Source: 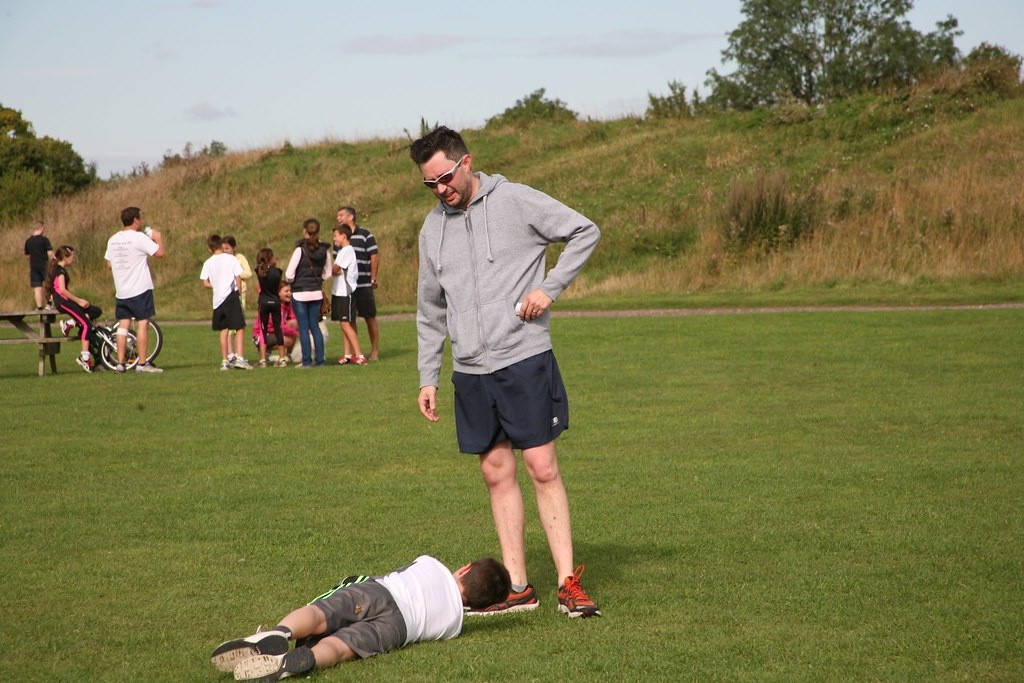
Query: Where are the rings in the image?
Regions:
[533,311,537,313]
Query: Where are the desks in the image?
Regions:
[0,309,61,337]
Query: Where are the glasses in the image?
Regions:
[423,155,464,188]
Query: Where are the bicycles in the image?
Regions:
[74,304,163,373]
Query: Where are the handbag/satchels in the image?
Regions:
[320,291,330,314]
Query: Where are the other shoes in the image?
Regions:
[294,363,312,368]
[273,358,287,367]
[315,360,325,366]
[45,304,53,310]
[220,363,233,370]
[255,359,266,368]
[229,356,237,366]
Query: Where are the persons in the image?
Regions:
[331,207,380,365]
[252,280,300,361]
[285,218,332,369]
[104,207,165,374]
[25,221,55,310]
[255,247,286,368]
[410,125,602,619]
[333,223,368,366]
[219,235,253,366]
[209,554,512,681]
[199,234,254,370]
[44,245,103,373]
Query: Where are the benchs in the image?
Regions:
[0,336,76,376]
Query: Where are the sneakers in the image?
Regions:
[135,361,163,373]
[59,320,73,337]
[338,355,352,365]
[76,354,92,373]
[234,647,315,683]
[116,363,126,373]
[465,584,539,616]
[350,354,367,365]
[234,358,253,369]
[557,565,602,618]
[210,625,291,673]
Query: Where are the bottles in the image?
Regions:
[143,225,152,238]
